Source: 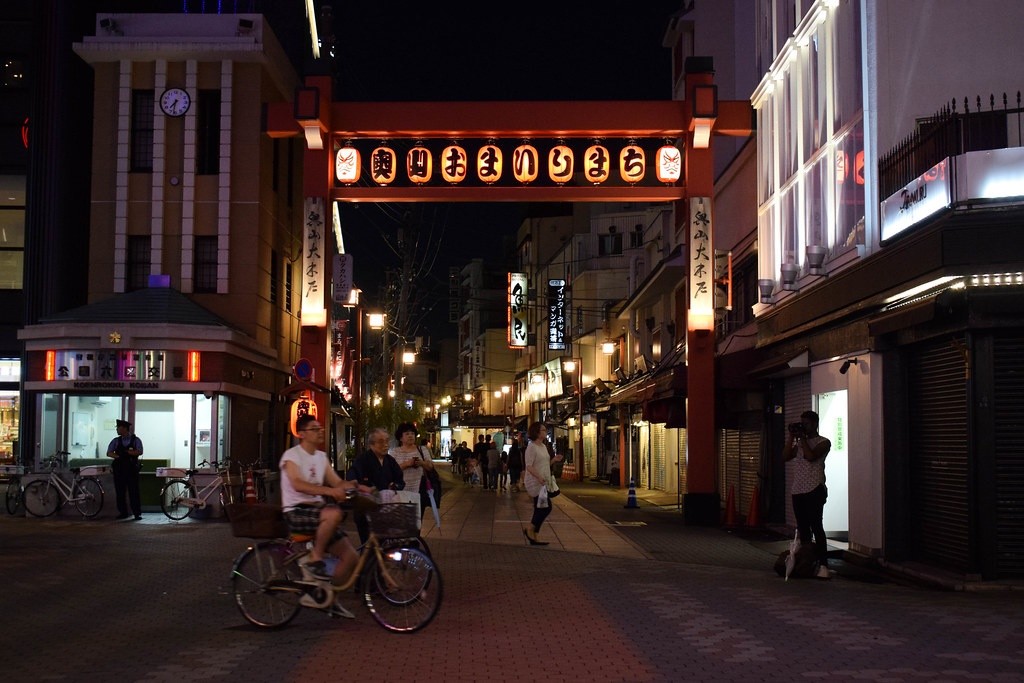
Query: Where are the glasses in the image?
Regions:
[302,427,324,432]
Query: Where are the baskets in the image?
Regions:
[369,502,419,539]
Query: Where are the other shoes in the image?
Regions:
[817,565,829,578]
[134,514,142,520]
[533,541,549,546]
[503,486,507,491]
[524,529,535,543]
[116,513,130,520]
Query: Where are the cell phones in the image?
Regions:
[129,448,133,451]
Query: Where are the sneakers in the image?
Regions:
[325,602,355,619]
[300,557,333,581]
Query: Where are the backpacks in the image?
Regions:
[788,541,818,578]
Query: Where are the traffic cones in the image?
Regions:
[623,478,641,508]
[561,462,579,483]
[244,468,257,503]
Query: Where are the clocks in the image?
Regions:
[159,88,191,117]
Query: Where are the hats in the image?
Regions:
[116,419,132,429]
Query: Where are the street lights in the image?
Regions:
[562,357,584,483]
[342,281,386,458]
[534,369,549,421]
[494,386,510,429]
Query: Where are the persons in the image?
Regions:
[390,422,432,565]
[781,411,831,578]
[280,414,375,618]
[107,419,143,521]
[523,422,562,545]
[448,435,522,492]
[346,428,406,596]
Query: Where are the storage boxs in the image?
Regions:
[0,465,24,474]
[80,466,110,475]
[156,467,187,477]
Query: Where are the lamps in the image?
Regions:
[100,18,117,35]
[758,279,775,303]
[806,246,829,277]
[781,263,800,291]
[599,334,617,356]
[839,357,857,374]
[236,19,254,37]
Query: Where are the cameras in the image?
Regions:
[787,422,806,434]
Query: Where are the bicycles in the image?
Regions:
[214,477,446,636]
[21,449,105,522]
[159,455,237,525]
[234,455,266,503]
[4,464,32,516]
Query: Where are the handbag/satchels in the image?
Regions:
[775,552,789,577]
[536,486,550,508]
[415,446,441,509]
[548,474,560,498]
[472,468,479,485]
